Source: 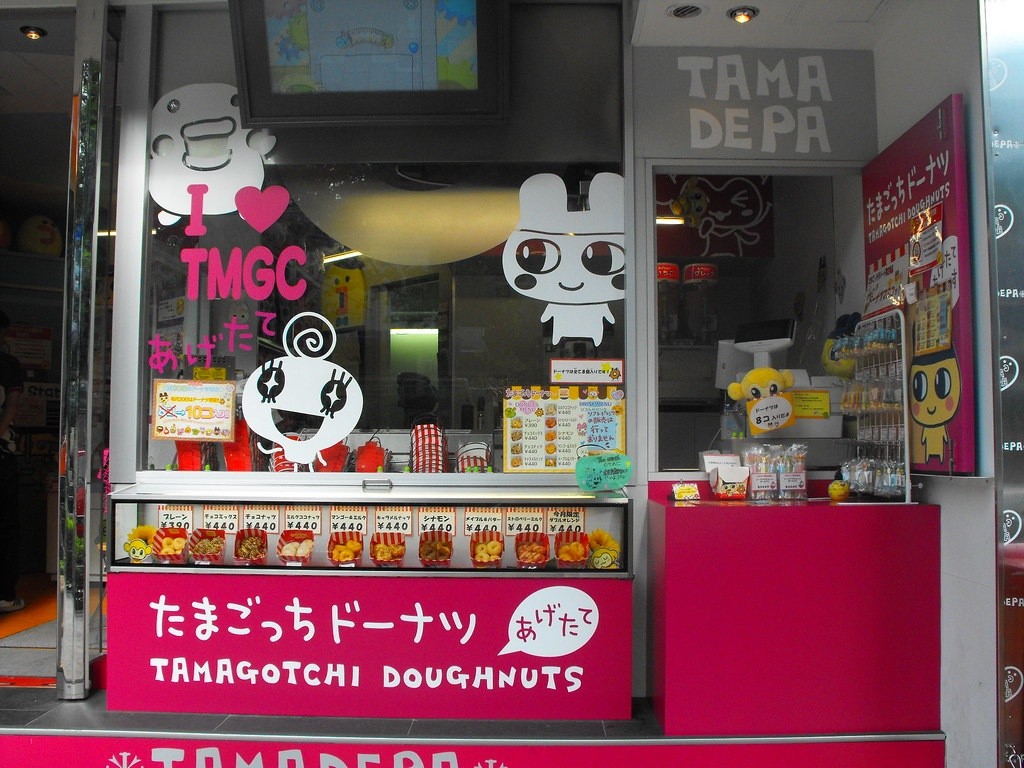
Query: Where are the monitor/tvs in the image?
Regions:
[716,340,788,390]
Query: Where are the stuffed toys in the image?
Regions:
[727,367,794,401]
[822,312,861,380]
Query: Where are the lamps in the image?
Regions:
[732,9,754,24]
[25,28,42,40]
[656,215,692,225]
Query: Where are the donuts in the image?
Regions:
[151,532,586,564]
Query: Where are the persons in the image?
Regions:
[396,372,437,425]
[0,311,27,613]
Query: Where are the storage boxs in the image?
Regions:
[326,531,365,567]
[370,531,406,567]
[554,531,590,569]
[710,466,750,500]
[188,528,227,565]
[514,531,551,568]
[469,530,505,569]
[233,528,268,567]
[276,528,314,565]
[418,529,453,568]
[150,527,189,564]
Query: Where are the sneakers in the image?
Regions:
[0,599,25,612]
